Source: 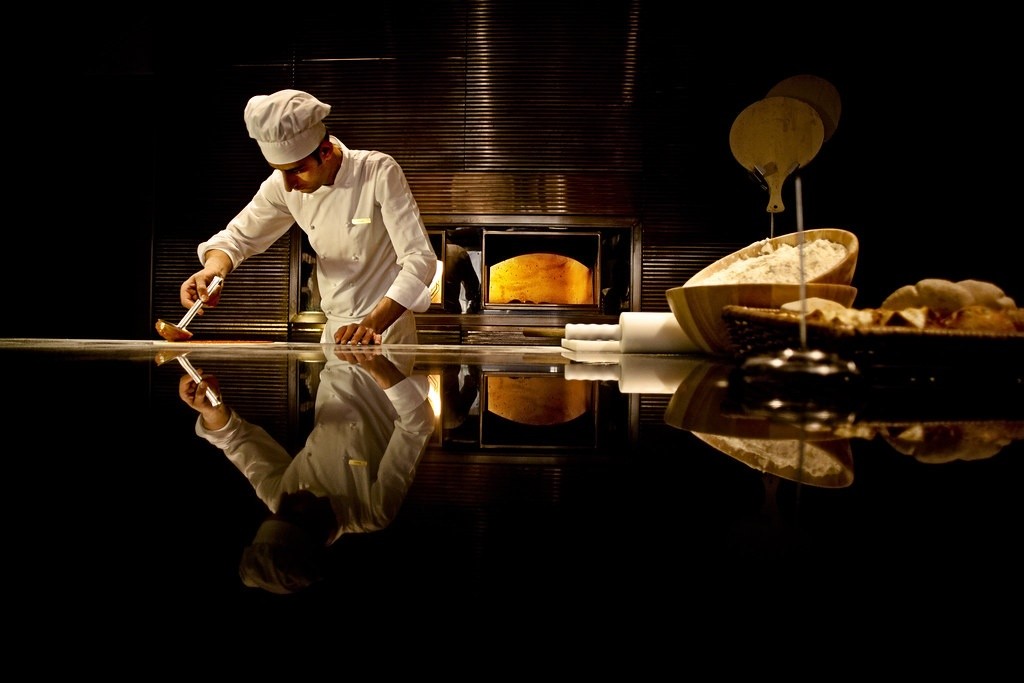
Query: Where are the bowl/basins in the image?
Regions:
[666,284,857,355]
[682,229,858,284]
[687,432,856,488]
[294,351,328,362]
[664,362,854,440]
[291,311,327,323]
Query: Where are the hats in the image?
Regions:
[239,520,319,596]
[244,88,331,165]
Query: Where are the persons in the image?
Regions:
[178,89,440,347]
[180,347,441,597]
[442,227,483,315]
[441,364,479,443]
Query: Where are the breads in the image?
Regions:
[779,278,1024,332]
